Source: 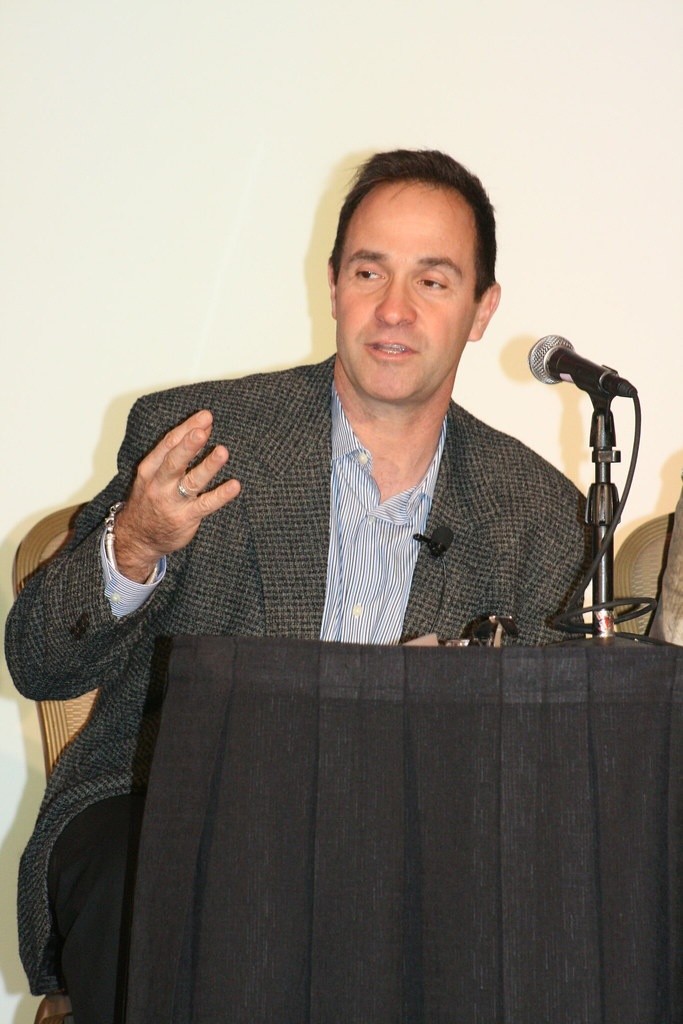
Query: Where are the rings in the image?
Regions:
[177,476,193,498]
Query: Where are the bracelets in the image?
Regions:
[106,502,158,585]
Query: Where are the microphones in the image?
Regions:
[528,334,638,399]
[413,527,454,558]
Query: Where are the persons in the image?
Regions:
[4,153,586,1024]
